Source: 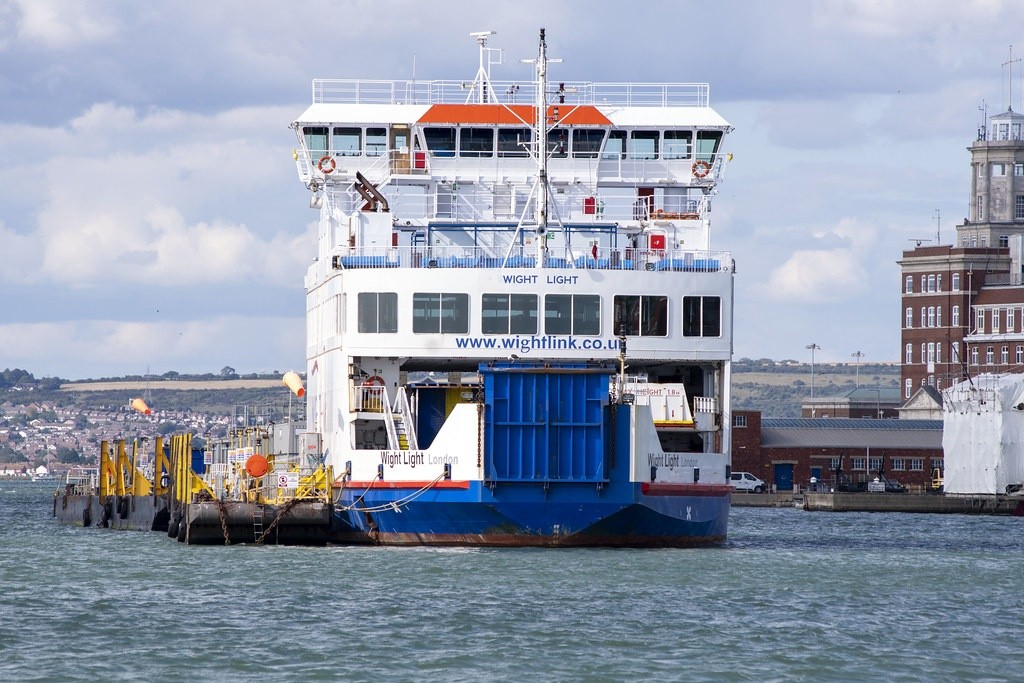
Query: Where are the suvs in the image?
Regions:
[930,467,945,494]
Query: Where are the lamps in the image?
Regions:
[622,394,635,405]
[645,262,655,271]
[460,392,473,402]
[429,260,437,268]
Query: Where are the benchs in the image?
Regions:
[341,256,721,271]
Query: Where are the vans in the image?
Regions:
[729,471,765,494]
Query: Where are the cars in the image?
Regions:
[880,478,906,492]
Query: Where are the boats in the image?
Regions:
[202,22,736,555]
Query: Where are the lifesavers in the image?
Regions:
[101,506,110,527]
[692,161,709,177]
[168,511,181,538]
[318,156,335,174]
[116,499,127,520]
[178,523,184,542]
[83,509,89,527]
[366,377,386,394]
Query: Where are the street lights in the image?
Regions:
[805,343,821,397]
[851,351,866,389]
